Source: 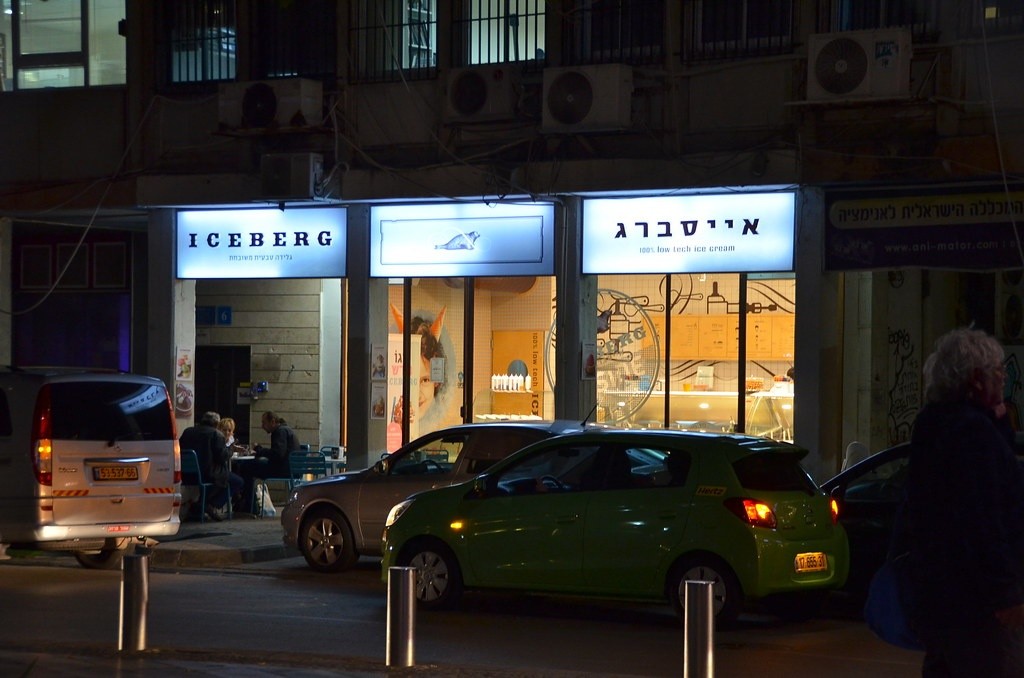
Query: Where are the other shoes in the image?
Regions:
[206,508,222,521]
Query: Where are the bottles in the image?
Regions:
[492,373,531,391]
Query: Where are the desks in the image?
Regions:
[225,454,254,470]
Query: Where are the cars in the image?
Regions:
[817,442,915,615]
[280,419,613,573]
[380,429,850,632]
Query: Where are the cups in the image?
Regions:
[683,384,691,391]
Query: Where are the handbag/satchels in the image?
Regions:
[256,484,276,516]
[864,552,926,650]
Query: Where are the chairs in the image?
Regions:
[181,448,233,525]
[289,450,327,491]
[249,445,310,518]
[321,446,349,476]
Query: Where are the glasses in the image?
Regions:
[992,364,1005,373]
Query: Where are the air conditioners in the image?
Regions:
[439,61,526,122]
[216,76,323,135]
[249,153,323,200]
[538,63,632,131]
[805,27,913,103]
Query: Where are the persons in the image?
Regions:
[180,411,244,521]
[890,327,1024,678]
[231,412,295,518]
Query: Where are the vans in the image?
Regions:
[1,363,183,571]
